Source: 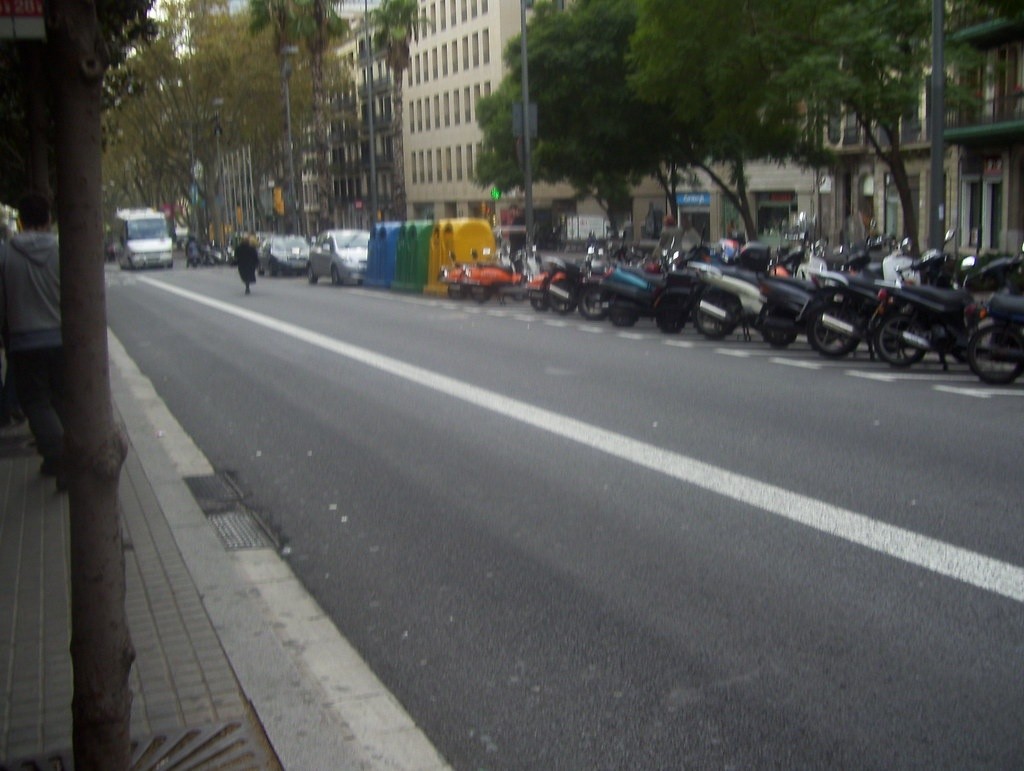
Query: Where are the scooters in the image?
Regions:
[176,235,237,268]
[433,203,1024,387]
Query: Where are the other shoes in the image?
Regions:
[245,288,250,294]
[40,454,70,487]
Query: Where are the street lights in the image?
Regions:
[279,44,300,234]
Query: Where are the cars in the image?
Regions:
[305,227,371,286]
[256,233,310,277]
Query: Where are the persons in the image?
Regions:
[185,235,211,268]
[726,220,736,239]
[653,216,683,257]
[236,236,259,294]
[0,195,71,494]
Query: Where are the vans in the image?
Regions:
[111,206,174,271]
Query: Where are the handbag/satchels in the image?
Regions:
[258,265,265,275]
